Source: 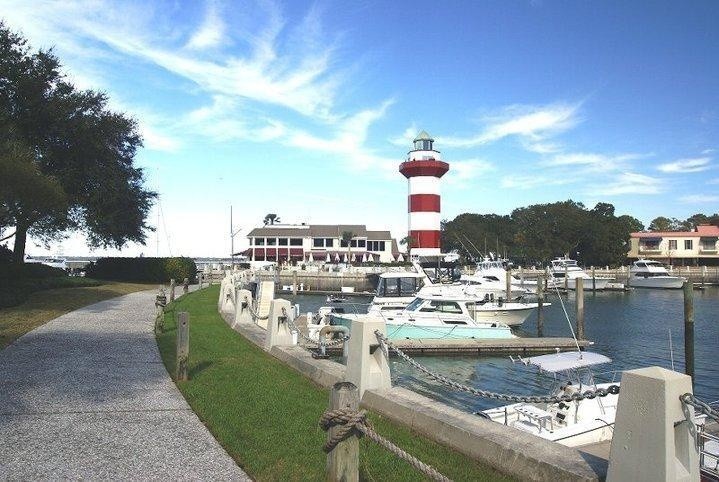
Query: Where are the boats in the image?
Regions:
[467,378,622,449]
[541,256,615,291]
[624,256,686,290]
[324,263,549,346]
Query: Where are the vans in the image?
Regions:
[42,256,69,272]
